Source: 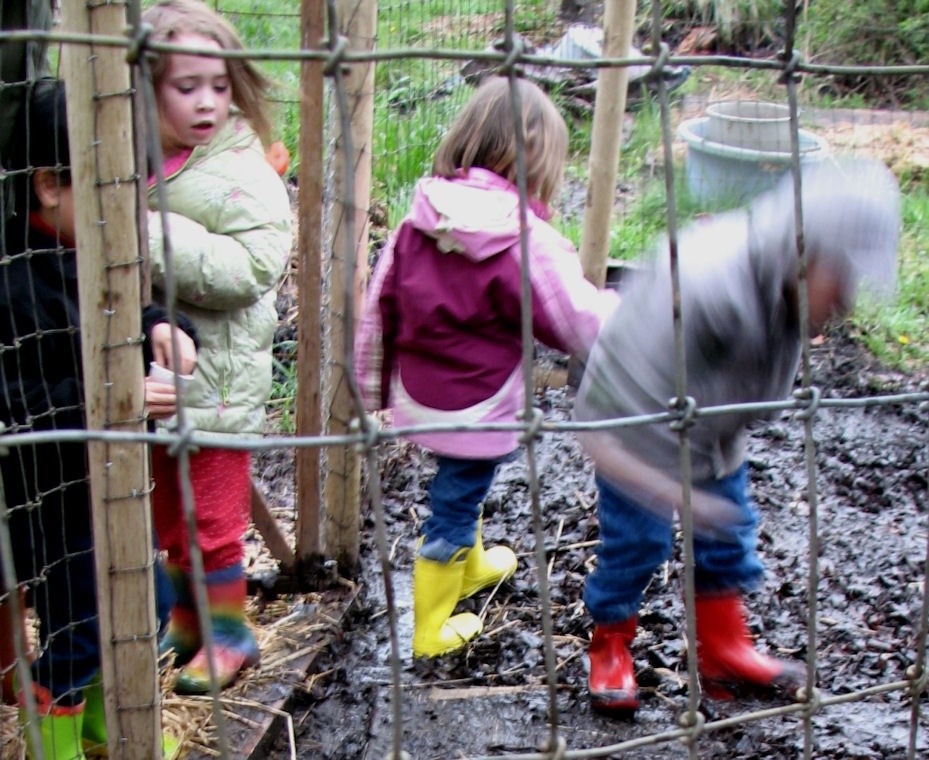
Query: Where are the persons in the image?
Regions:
[572,159,901,718]
[0,0,296,760]
[357,74,620,659]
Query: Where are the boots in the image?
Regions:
[176,563,262,694]
[412,554,483,660]
[155,549,202,668]
[685,589,811,702]
[418,518,520,601]
[16,696,82,759]
[587,614,642,710]
[82,677,180,760]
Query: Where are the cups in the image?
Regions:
[149,361,195,424]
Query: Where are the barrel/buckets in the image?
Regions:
[706,97,805,151]
[677,117,829,212]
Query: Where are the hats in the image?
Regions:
[802,158,901,299]
